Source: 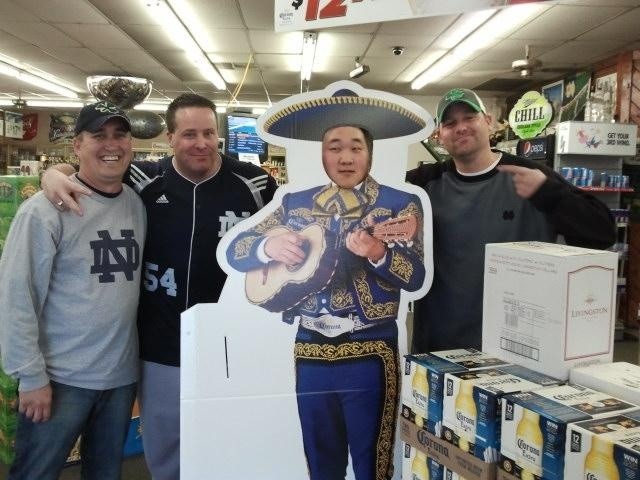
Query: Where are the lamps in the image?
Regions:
[298,32,317,82]
[0,52,83,101]
[410,3,555,92]
[136,0,228,92]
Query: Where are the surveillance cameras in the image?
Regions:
[349,65,370,78]
[392,47,404,56]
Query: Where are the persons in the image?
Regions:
[0,100,148,480]
[42,93,275,480]
[213,79,438,480]
[403,86,621,356]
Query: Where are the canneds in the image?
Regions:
[561,166,630,188]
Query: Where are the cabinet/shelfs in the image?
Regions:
[509,121,636,342]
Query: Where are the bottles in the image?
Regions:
[410,449,430,480]
[516,408,543,478]
[411,364,430,421]
[455,380,477,445]
[583,434,620,479]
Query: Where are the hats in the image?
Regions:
[263,86,427,143]
[73,101,133,137]
[436,87,485,122]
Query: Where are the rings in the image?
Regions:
[57,200,64,207]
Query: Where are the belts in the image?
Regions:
[298,311,377,340]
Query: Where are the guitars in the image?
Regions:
[245,216,417,313]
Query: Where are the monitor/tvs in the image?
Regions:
[226,113,267,159]
